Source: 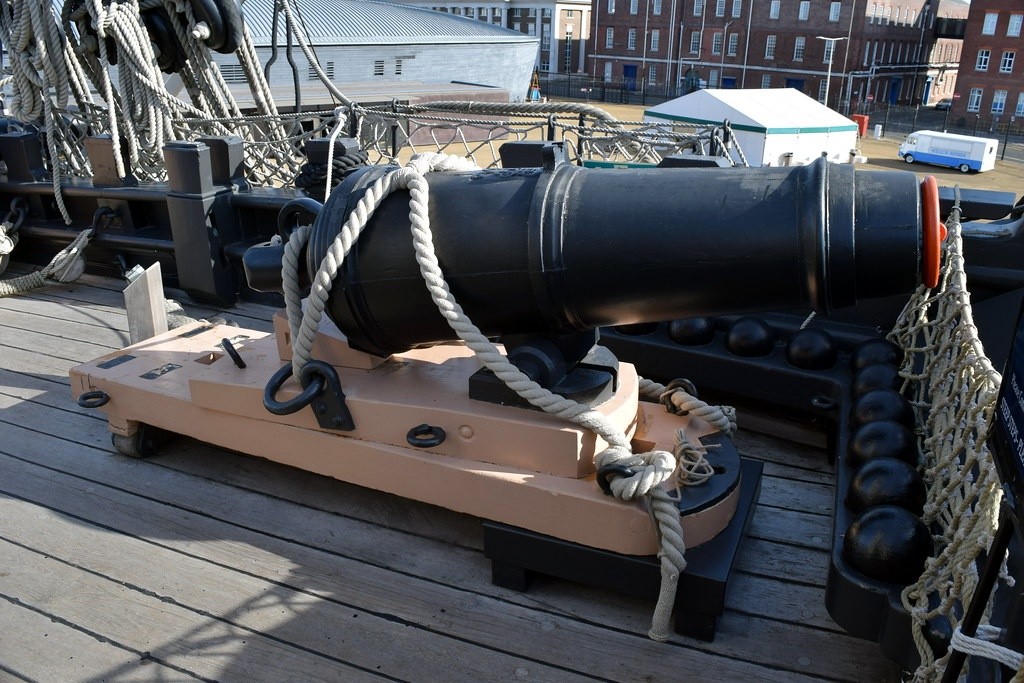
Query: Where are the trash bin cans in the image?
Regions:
[852,113,869,137]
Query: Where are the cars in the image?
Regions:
[935,98,951,111]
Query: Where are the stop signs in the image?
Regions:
[954,93,960,101]
[867,94,872,101]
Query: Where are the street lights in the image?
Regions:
[719,19,734,89]
[815,36,849,106]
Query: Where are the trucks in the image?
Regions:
[898,130,999,173]
